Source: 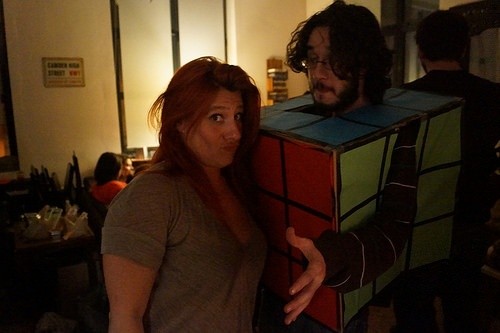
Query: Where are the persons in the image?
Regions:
[91,152,129,206]
[392,10,500,333]
[284,0,421,333]
[102,57,266,333]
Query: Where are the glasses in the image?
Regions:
[300,54,339,73]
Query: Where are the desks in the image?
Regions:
[0,201,104,312]
